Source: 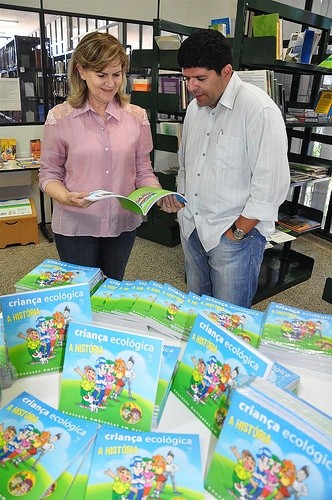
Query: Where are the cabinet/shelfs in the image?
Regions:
[0,1,332,306]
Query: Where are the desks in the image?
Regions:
[0,153,53,242]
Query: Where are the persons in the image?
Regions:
[37,32,185,282]
[175,30,291,309]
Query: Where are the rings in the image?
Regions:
[167,204,170,208]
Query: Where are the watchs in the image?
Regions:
[231,223,246,240]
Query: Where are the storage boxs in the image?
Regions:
[0,197,38,248]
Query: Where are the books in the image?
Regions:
[0,13,332,249]
[84,187,188,216]
[0,259,332,500]
